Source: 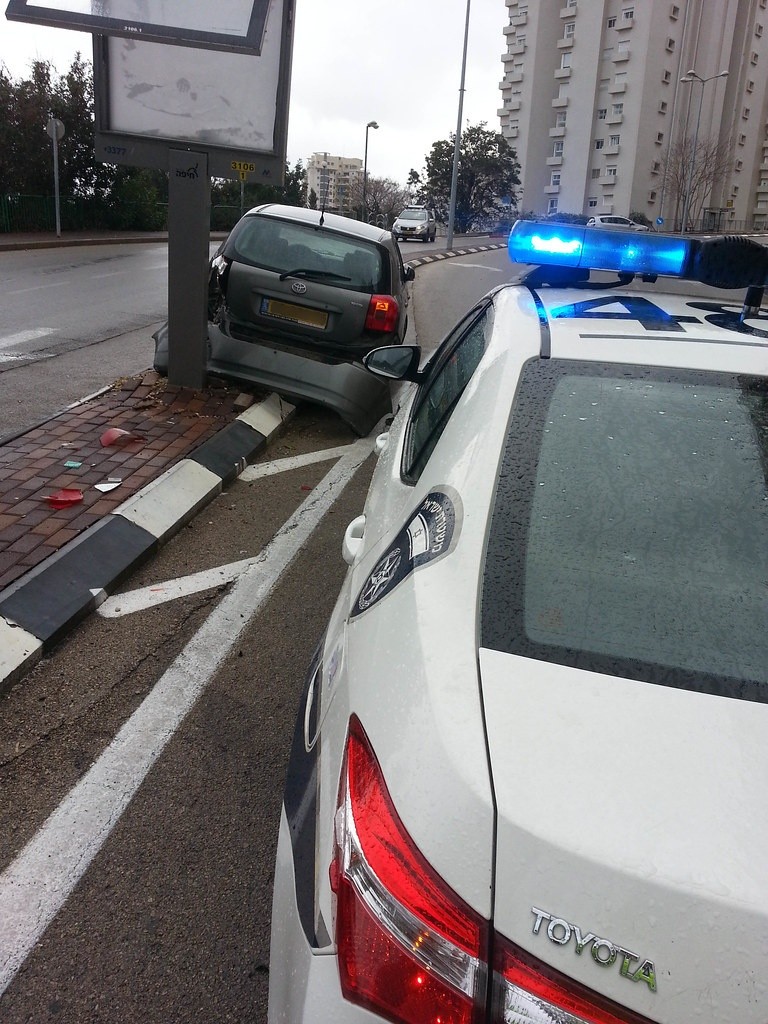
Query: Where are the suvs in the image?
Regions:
[586,215,650,232]
[392,203,438,242]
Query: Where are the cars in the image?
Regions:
[259,215,767,1024]
[151,203,416,436]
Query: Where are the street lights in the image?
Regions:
[363,120,379,202]
[679,69,730,236]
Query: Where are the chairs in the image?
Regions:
[343,250,377,276]
[266,237,310,268]
